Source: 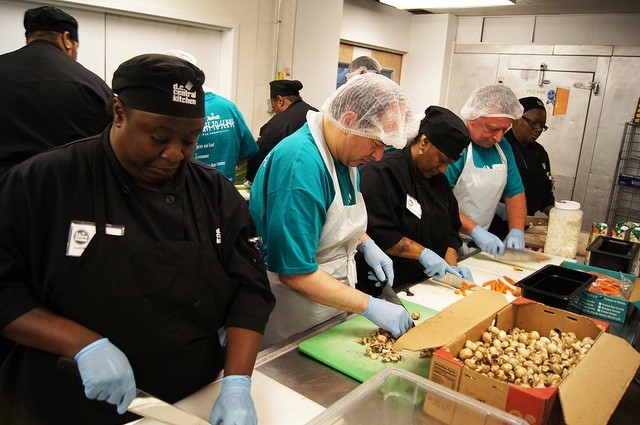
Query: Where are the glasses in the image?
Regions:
[522,116,548,133]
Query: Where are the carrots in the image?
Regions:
[455,276,521,297]
[587,272,623,296]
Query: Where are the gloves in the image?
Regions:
[359,239,394,287]
[470,224,506,257]
[506,228,526,251]
[359,295,413,339]
[207,375,258,425]
[73,337,138,414]
[451,265,473,280]
[419,247,462,281]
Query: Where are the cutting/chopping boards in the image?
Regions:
[396,265,519,312]
[122,368,344,425]
[457,247,577,281]
[524,225,590,256]
[298,297,440,383]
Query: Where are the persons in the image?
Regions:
[159,48,259,185]
[445,83,529,256]
[358,104,476,298]
[344,55,383,81]
[248,72,415,352]
[0,5,114,176]
[503,97,556,230]
[0,53,277,424]
[243,80,321,189]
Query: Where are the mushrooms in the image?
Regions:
[453,326,594,388]
[352,311,434,363]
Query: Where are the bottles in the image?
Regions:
[544,200,583,260]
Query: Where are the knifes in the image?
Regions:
[416,260,481,291]
[467,241,551,262]
[369,266,415,328]
[57,356,211,425]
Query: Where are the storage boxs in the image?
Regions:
[585,234,636,273]
[560,261,636,323]
[393,288,637,424]
[516,264,598,308]
[304,365,531,425]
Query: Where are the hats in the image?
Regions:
[518,96,546,112]
[112,54,205,118]
[421,105,472,161]
[269,80,303,96]
[23,5,79,43]
[163,49,200,67]
